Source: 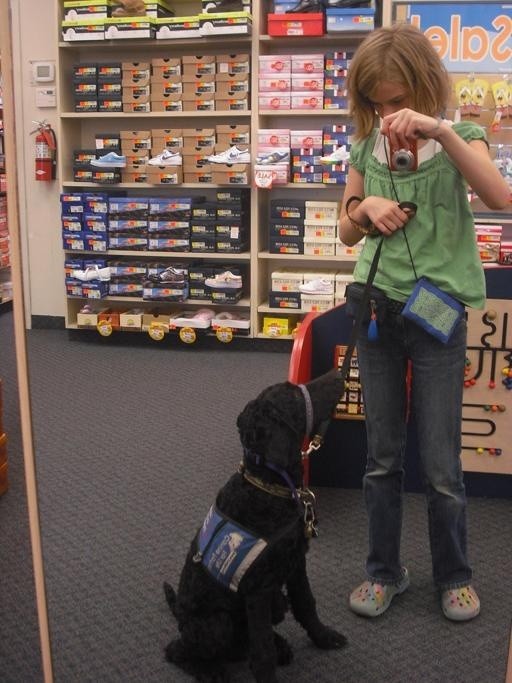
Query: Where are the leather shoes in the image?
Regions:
[285,0,320,13]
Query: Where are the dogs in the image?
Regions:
[162,367,348,683]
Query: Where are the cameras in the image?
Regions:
[389,139,418,172]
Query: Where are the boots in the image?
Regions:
[111,0,146,17]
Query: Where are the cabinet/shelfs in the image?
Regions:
[55,0,393,339]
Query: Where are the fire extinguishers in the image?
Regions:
[30,120,57,181]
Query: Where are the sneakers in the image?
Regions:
[80,305,93,314]
[208,145,251,164]
[89,152,127,168]
[257,152,290,165]
[149,267,184,284]
[299,279,334,294]
[320,144,351,161]
[208,0,243,12]
[147,150,182,166]
[205,271,242,289]
[74,265,111,281]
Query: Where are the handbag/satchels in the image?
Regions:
[344,284,386,341]
[402,279,464,344]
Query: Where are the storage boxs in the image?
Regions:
[475,224,511,266]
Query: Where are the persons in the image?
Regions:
[334,20,510,623]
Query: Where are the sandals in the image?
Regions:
[350,567,410,617]
[441,585,481,621]
